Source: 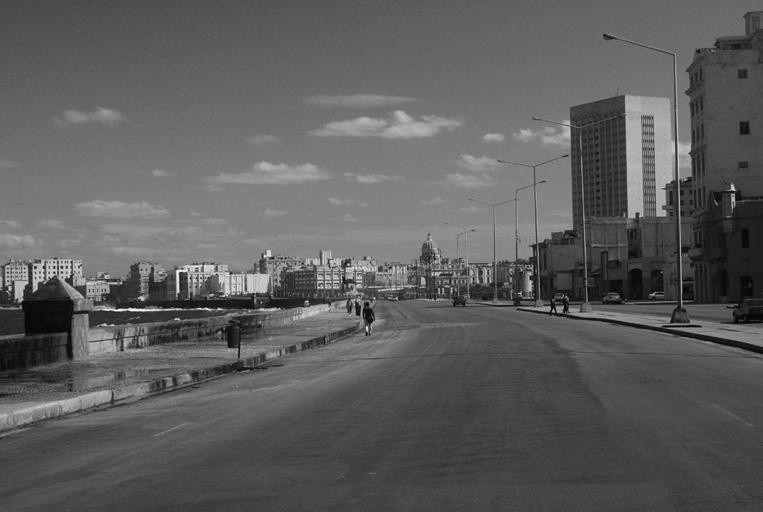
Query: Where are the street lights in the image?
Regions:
[603,33,683,307]
[533,115,626,303]
[443,154,569,299]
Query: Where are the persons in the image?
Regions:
[346,297,353,316]
[353,298,363,320]
[562,297,570,318]
[363,302,376,336]
[549,296,559,316]
[562,292,571,313]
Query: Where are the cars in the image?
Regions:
[602,292,621,304]
[733,299,762,323]
[648,291,664,301]
[553,293,569,303]
[454,296,465,307]
